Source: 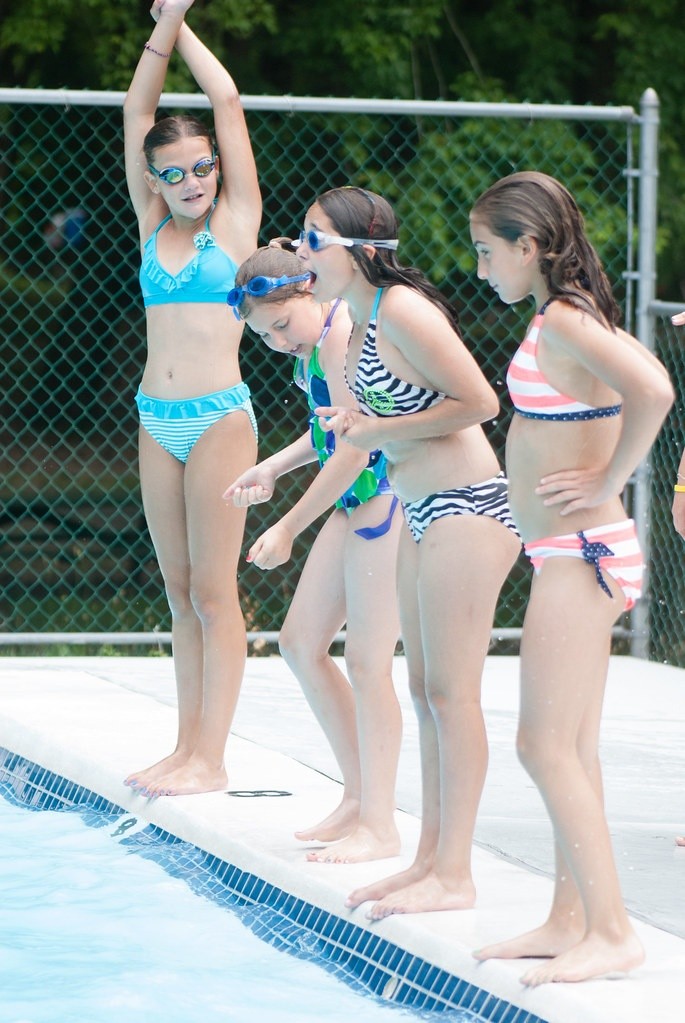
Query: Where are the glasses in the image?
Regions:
[226,276,288,305]
[148,151,215,185]
[300,230,354,250]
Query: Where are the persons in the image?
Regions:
[296,184,520,923]
[671,446,685,541]
[121,0,264,796]
[221,239,403,860]
[468,171,675,987]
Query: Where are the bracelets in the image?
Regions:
[144,42,171,58]
[674,473,685,493]
